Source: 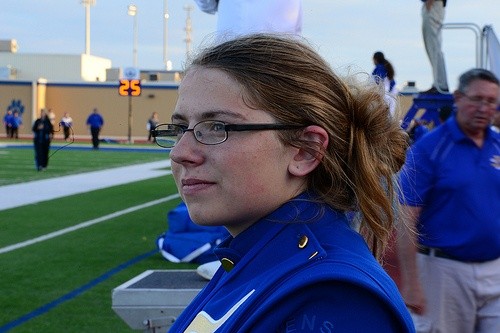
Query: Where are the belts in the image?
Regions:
[416,247,465,263]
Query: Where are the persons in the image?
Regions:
[3,106,160,172]
[370,0,500,333]
[151,34,418,333]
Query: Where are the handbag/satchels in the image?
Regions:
[155,201,232,264]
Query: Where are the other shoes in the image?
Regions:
[419,86,448,94]
[196,260,223,280]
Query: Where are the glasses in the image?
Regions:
[150,120,311,148]
[463,93,500,108]
